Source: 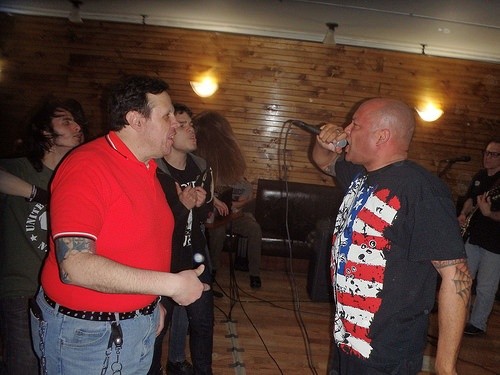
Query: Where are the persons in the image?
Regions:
[30,74,210,375]
[165,112,261,375]
[0,100,84,375]
[460,138,500,335]
[147,102,214,375]
[313,98,472,375]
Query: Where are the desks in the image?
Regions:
[204,206,243,228]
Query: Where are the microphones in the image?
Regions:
[290,120,347,150]
[440,156,472,163]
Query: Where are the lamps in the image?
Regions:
[414,106,444,123]
[190,80,218,99]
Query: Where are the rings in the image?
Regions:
[336,129,340,132]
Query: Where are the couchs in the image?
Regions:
[241,177,344,270]
